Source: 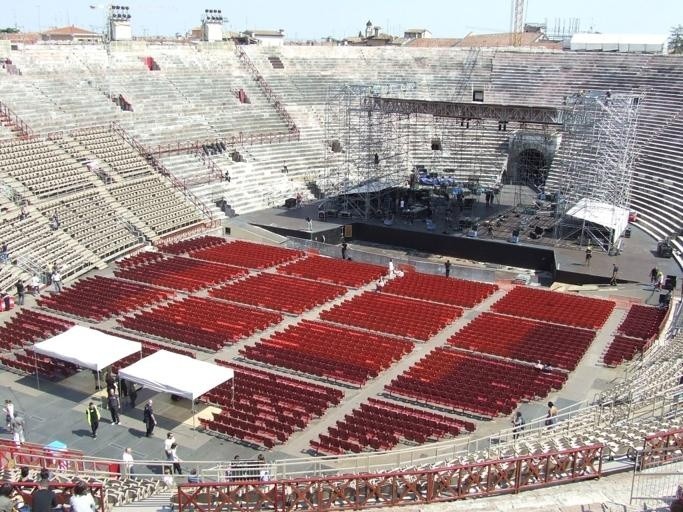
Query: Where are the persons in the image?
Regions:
[609,263,617,284]
[35,467,57,490]
[105,387,122,425]
[388,259,394,276]
[534,359,543,370]
[187,467,204,482]
[143,398,156,437]
[164,432,175,459]
[169,442,182,474]
[581,90,586,97]
[648,267,657,287]
[376,276,384,287]
[48,214,57,230]
[9,409,26,445]
[225,455,245,482]
[30,273,39,295]
[50,270,62,293]
[106,374,137,408]
[485,191,490,208]
[0,483,30,511]
[547,401,557,434]
[282,160,288,174]
[93,366,105,389]
[31,479,63,511]
[604,88,613,106]
[84,401,101,440]
[340,241,347,259]
[305,216,312,231]
[17,466,34,491]
[122,446,135,479]
[295,193,300,208]
[584,246,592,263]
[14,280,25,304]
[0,241,7,265]
[543,360,551,370]
[257,453,271,480]
[224,170,230,182]
[2,397,15,434]
[489,193,494,207]
[657,272,663,292]
[511,411,525,439]
[574,90,582,104]
[0,458,16,482]
[444,260,451,280]
[68,480,96,512]
[51,262,58,273]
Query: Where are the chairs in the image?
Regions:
[0,42,683,512]
